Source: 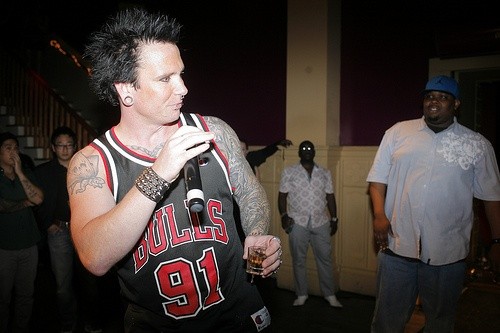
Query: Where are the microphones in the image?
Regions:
[184,146,205,213]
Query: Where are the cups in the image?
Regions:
[247,245,267,275]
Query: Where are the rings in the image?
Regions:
[269,237,277,243]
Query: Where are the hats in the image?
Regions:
[425,75,459,98]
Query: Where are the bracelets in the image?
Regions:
[135,168,170,202]
[249,232,263,236]
[331,218,338,222]
[282,212,286,216]
[490,239,500,243]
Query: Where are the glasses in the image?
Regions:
[54,143,75,148]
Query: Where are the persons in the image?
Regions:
[37,126,103,333]
[66,9,282,333]
[366,76,500,333]
[0,131,44,333]
[239,139,293,174]
[277,141,344,308]
